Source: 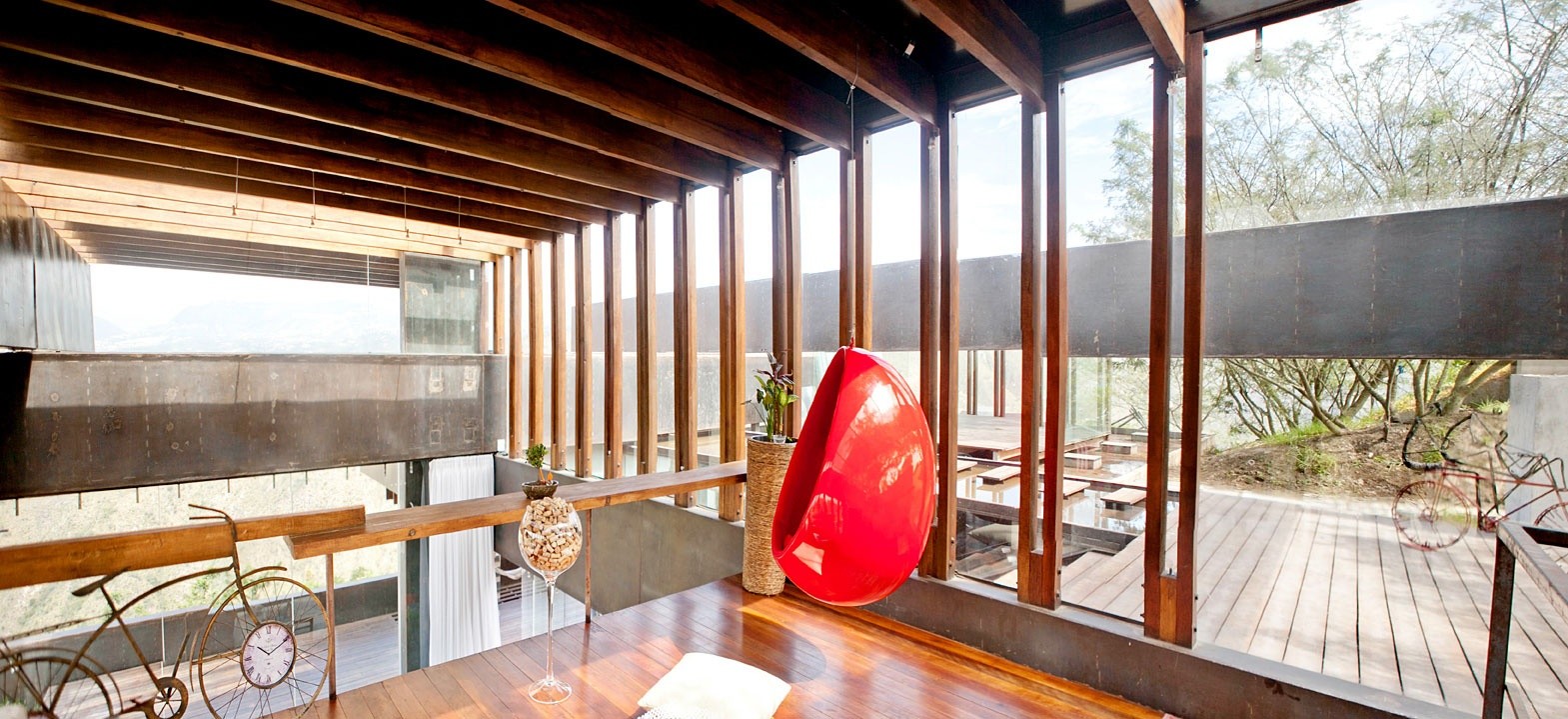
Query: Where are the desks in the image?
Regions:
[260,571,1178,719]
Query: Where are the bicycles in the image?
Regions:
[1391,415,1568,575]
[0,502,332,719]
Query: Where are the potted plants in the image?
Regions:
[740,347,798,597]
[521,444,559,501]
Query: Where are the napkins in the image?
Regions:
[637,654,791,719]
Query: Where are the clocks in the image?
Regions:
[240,620,298,689]
